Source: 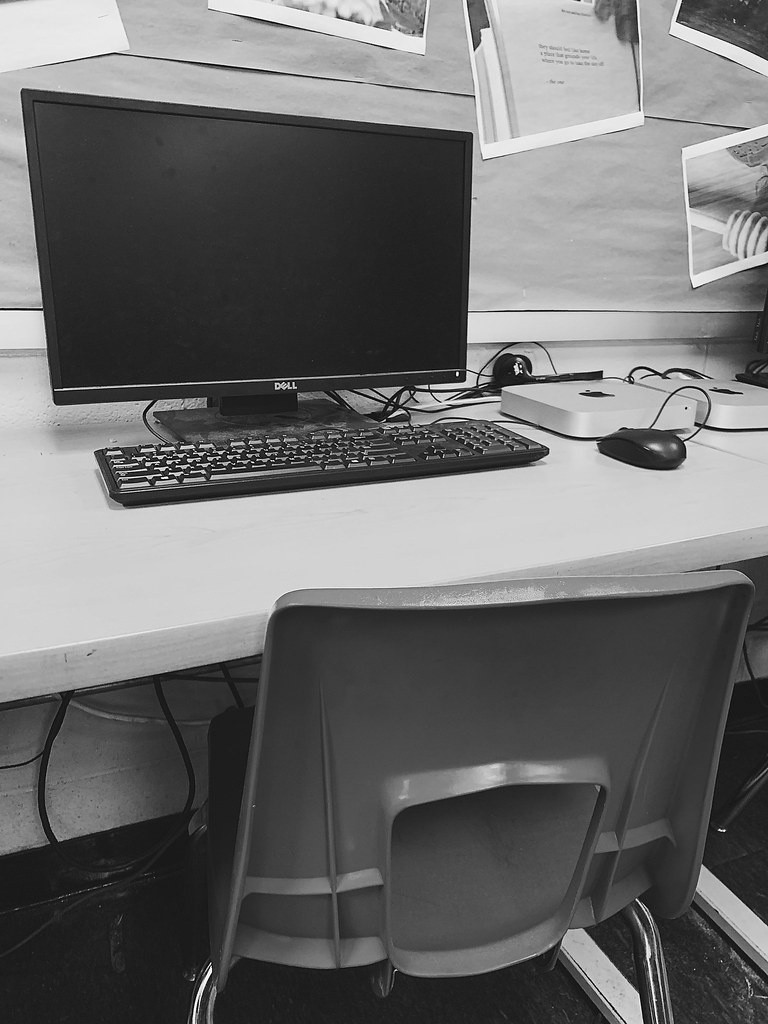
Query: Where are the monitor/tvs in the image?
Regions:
[22,88,474,406]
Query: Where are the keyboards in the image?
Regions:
[95,419,551,506]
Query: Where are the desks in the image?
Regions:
[0,353,768,715]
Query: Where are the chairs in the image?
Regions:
[179,570,757,1023]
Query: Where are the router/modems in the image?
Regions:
[500,381,768,437]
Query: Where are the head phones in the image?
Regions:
[493,353,603,384]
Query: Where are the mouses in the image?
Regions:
[595,427,686,470]
